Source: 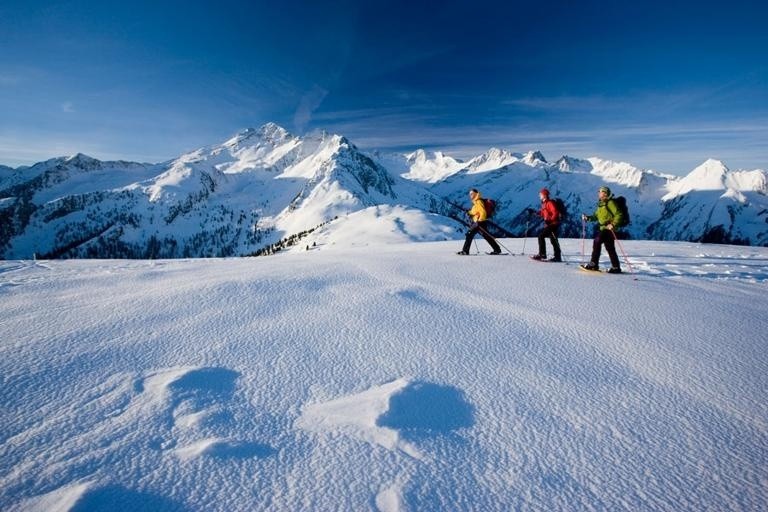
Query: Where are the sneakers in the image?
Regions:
[581,262,599,272]
[489,248,502,255]
[457,250,469,255]
[531,253,547,261]
[550,255,562,262]
[607,267,622,274]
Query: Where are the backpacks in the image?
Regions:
[605,193,630,228]
[476,198,497,219]
[547,197,566,222]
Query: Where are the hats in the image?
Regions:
[539,188,550,196]
[598,185,611,197]
[470,188,478,194]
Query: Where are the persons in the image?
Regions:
[529,188,566,263]
[456,188,502,255]
[580,185,623,274]
